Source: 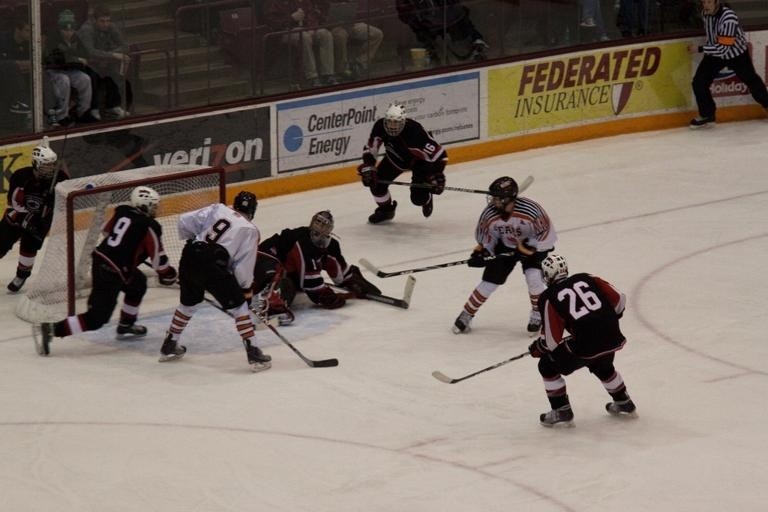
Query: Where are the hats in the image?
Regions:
[57,9,75,31]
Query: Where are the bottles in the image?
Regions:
[297,8,305,27]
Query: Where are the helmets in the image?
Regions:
[383,104,405,137]
[234,191,257,222]
[541,254,568,287]
[310,211,334,241]
[130,186,161,214]
[486,177,518,210]
[32,144,57,178]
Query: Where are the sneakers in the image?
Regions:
[691,115,715,125]
[47,107,130,128]
[306,70,358,88]
[9,103,32,114]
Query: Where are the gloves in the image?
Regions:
[160,267,176,285]
[358,162,378,186]
[244,284,253,305]
[21,212,46,231]
[42,190,55,207]
[427,172,445,194]
[468,243,491,267]
[528,337,551,358]
[514,237,536,258]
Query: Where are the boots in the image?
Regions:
[41,321,61,354]
[117,321,147,335]
[160,331,186,355]
[527,309,542,332]
[606,387,635,413]
[369,200,396,222]
[243,337,271,363]
[422,193,433,216]
[540,394,574,424]
[8,268,32,291]
[454,309,474,331]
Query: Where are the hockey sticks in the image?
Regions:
[248,306,338,368]
[323,275,416,309]
[359,251,516,279]
[431,334,574,385]
[378,175,535,195]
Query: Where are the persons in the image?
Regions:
[356,98,449,226]
[573,1,680,42]
[454,176,556,335]
[159,191,272,365]
[395,1,491,68]
[40,185,178,356]
[168,0,382,92]
[1,0,133,128]
[253,208,382,322]
[1,145,69,294]
[531,254,636,427]
[684,2,767,128]
